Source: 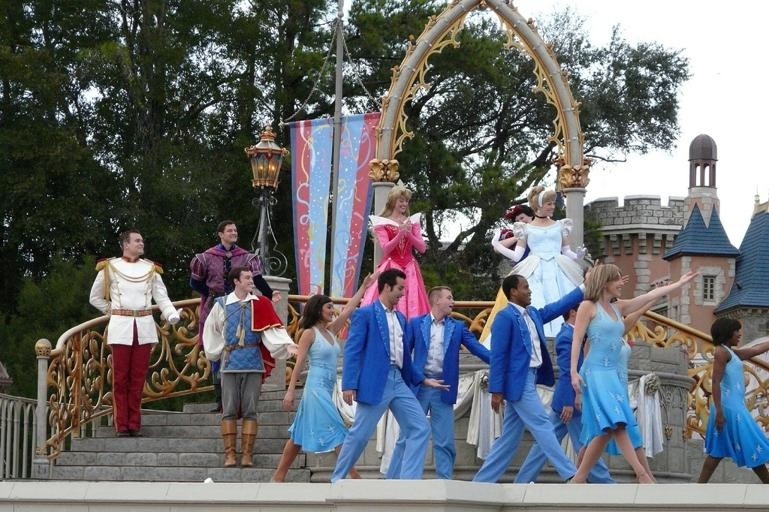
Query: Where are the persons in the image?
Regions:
[359,186,429,321]
[473,260,598,483]
[479,204,536,352]
[203,266,299,466]
[699,318,769,483]
[516,302,616,483]
[269,270,389,482]
[90,229,183,439]
[330,267,452,481]
[386,284,491,480]
[570,265,701,483]
[577,337,657,483]
[189,220,283,412]
[484,184,588,350]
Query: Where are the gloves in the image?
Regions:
[561,245,585,261]
[492,229,525,263]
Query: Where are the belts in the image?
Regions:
[113,309,153,317]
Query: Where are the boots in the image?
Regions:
[241,420,256,466]
[222,421,236,466]
[210,384,221,412]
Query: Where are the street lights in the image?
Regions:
[244,123,289,277]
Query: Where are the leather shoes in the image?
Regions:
[117,431,143,437]
